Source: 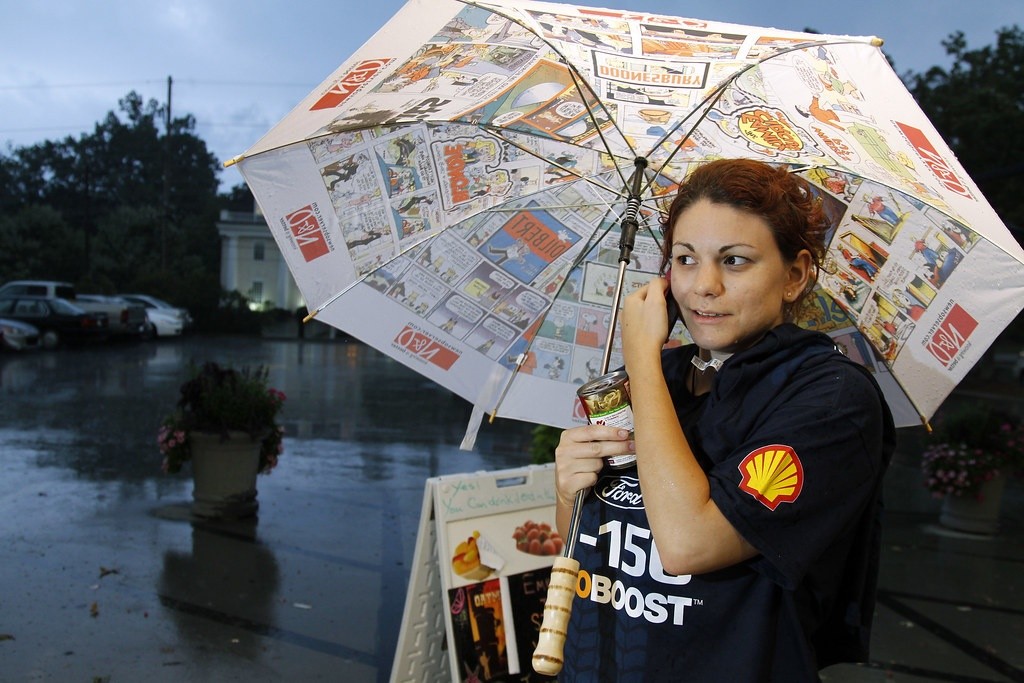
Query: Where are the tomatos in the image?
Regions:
[512,520,564,556]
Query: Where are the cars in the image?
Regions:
[0,293,110,349]
[0,319,42,353]
[117,293,193,344]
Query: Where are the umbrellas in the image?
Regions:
[224,0,1024,678]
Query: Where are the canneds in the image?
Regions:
[576,372,637,470]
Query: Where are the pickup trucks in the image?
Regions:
[0,280,147,343]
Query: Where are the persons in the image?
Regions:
[550,155,888,682]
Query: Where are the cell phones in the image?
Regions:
[661,265,679,345]
